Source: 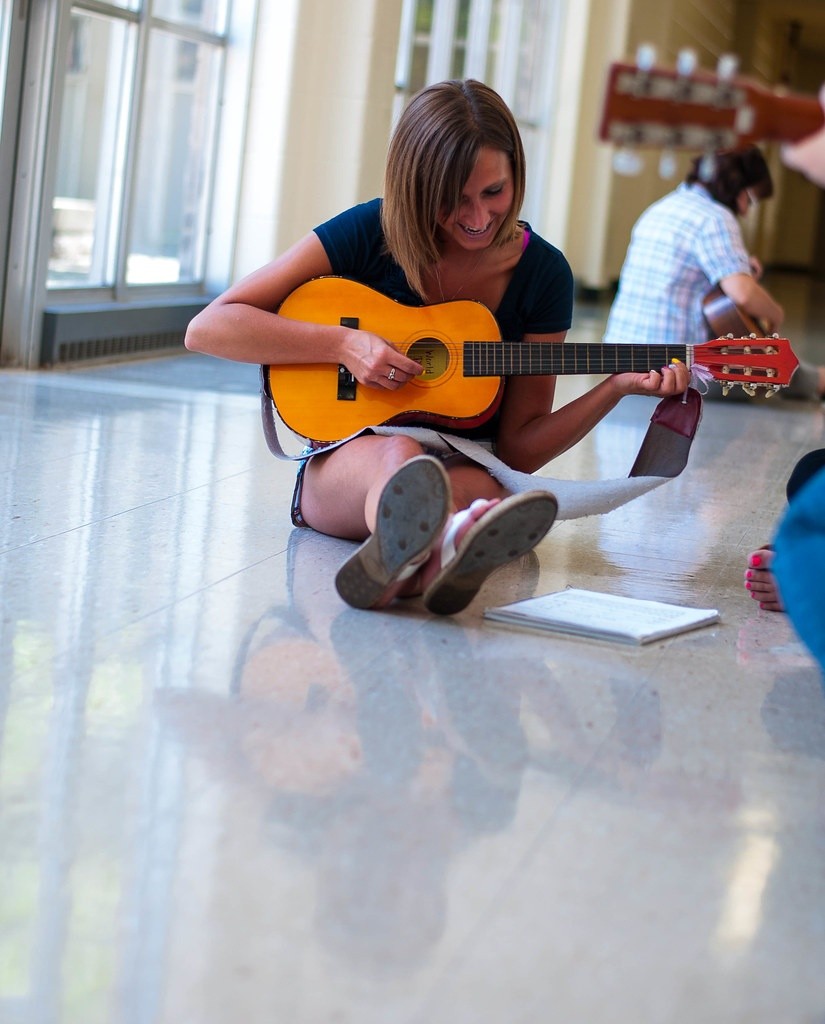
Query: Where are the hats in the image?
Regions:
[690,142,773,198]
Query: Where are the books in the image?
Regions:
[484,589,719,648]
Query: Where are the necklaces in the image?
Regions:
[432,244,492,303]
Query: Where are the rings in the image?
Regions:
[387,367,396,379]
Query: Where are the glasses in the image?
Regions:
[746,188,757,208]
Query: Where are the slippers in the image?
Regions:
[335,455,558,617]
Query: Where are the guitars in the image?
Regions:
[262,273,803,449]
[700,283,769,338]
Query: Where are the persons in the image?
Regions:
[745,449,825,611]
[603,145,825,400]
[183,77,692,616]
